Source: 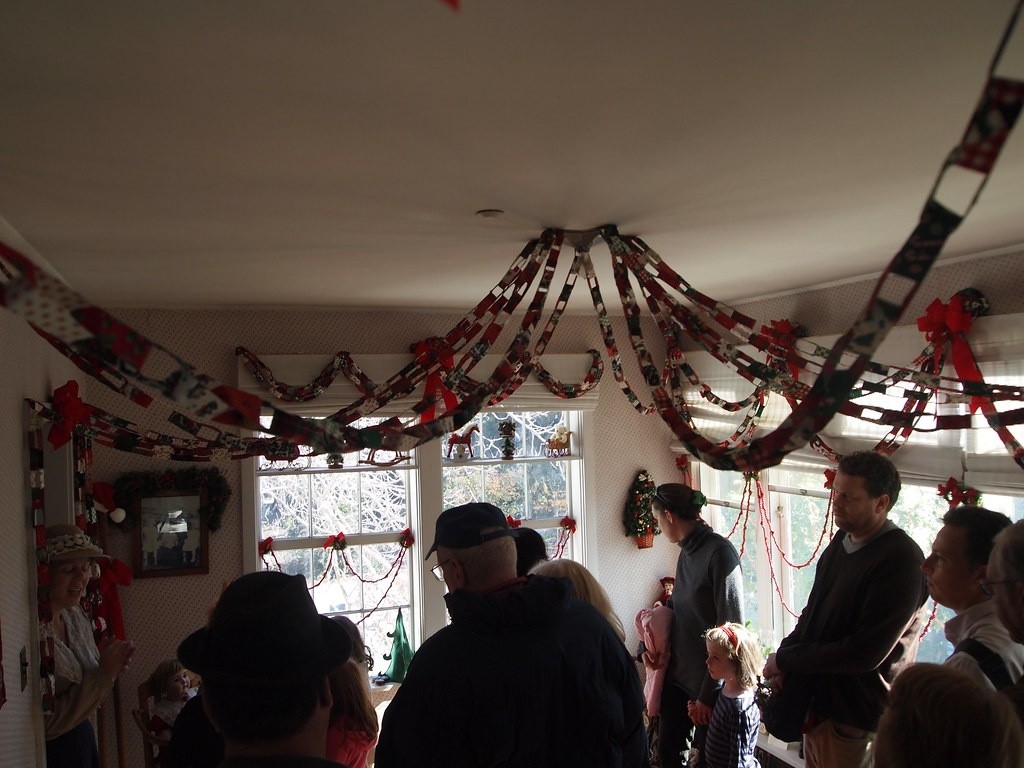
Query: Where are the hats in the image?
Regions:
[45,522,113,562]
[425,501,514,564]
[176,571,354,681]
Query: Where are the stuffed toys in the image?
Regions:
[658,577,676,607]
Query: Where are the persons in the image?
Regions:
[148,659,200,768]
[141,508,201,568]
[147,450,1024,768]
[36,523,139,768]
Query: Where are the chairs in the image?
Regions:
[132,665,201,768]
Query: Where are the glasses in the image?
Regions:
[431,559,450,583]
[652,485,670,508]
[978,577,1021,595]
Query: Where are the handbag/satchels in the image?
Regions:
[761,671,815,743]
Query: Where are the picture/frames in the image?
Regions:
[132,487,211,576]
